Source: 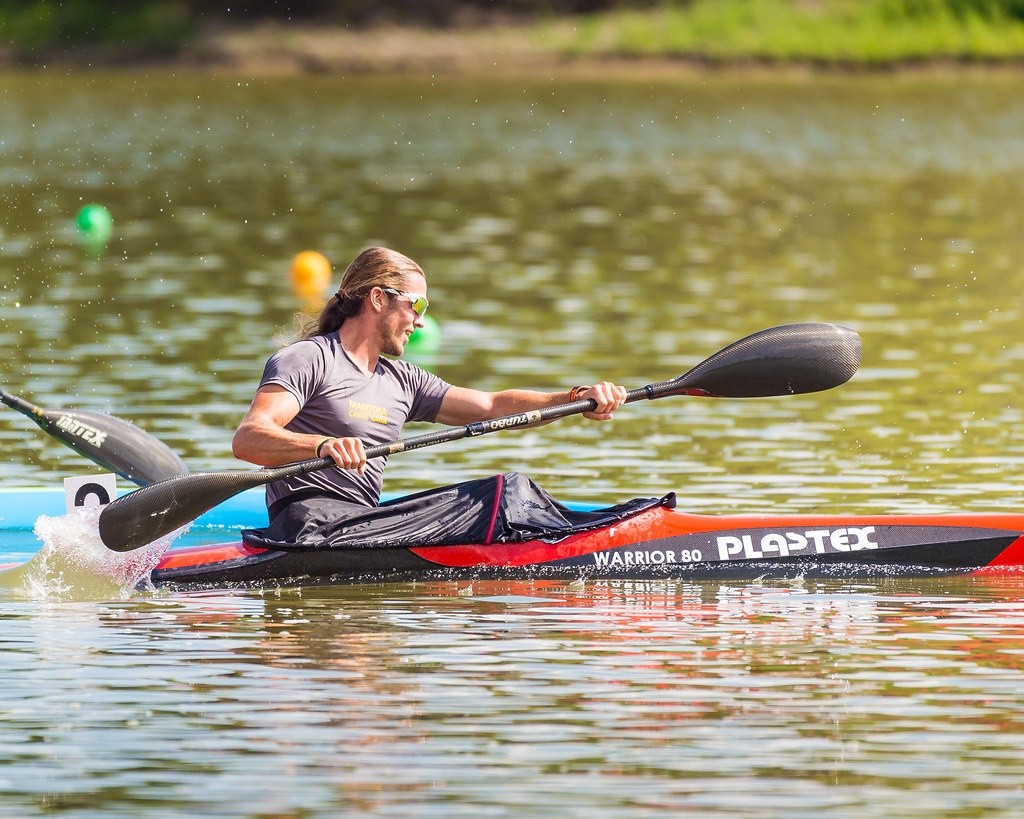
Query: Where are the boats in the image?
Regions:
[0,487,620,554]
[109,488,1023,591]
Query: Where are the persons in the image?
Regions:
[232,247,627,541]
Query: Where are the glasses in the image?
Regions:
[363,288,429,318]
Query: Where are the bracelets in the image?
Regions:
[570,386,591,402]
[314,436,336,458]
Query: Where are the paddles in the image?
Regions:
[0,392,191,489]
[97,322,863,553]
